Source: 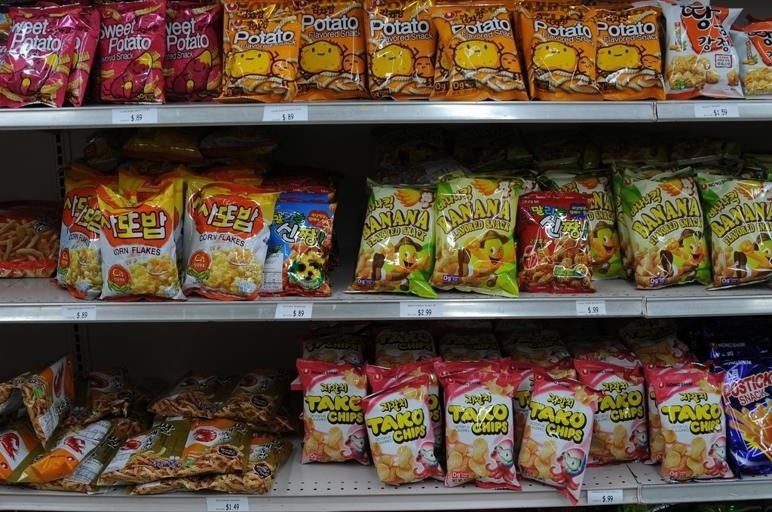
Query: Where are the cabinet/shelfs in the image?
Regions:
[0,0,771,511]
